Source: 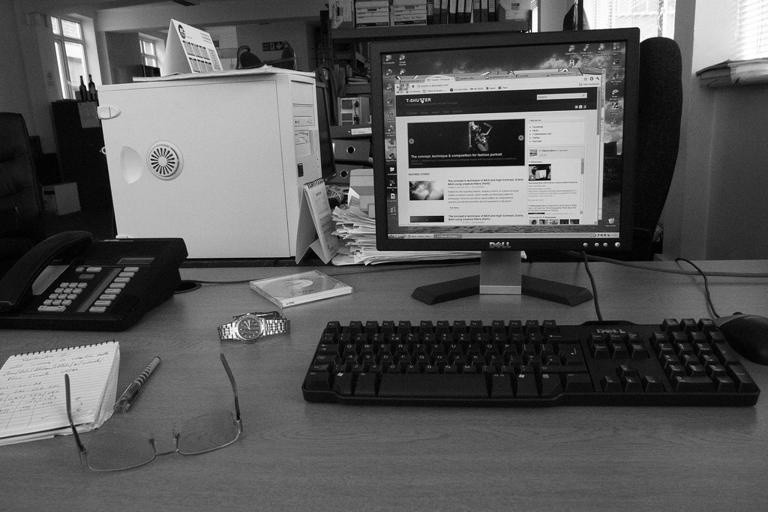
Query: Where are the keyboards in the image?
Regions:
[301,316,761,407]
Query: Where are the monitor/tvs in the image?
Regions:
[369,27,641,308]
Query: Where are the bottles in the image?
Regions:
[79,75,97,102]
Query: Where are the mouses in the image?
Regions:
[714,310,768,366]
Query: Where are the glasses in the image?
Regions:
[64,351,246,478]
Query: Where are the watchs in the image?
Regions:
[218,311,291,341]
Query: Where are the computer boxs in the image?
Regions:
[97,72,322,261]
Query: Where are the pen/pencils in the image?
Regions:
[113,356,161,413]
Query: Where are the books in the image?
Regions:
[250,269,353,309]
[302,179,482,266]
[0,341,120,446]
[133,64,316,82]
[696,58,767,81]
[348,77,368,84]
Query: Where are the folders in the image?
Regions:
[433,1,496,23]
[328,124,374,184]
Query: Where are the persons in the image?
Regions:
[472,125,493,151]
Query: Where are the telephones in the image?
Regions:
[0,230,188,332]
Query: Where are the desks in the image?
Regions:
[1,252,768,512]
